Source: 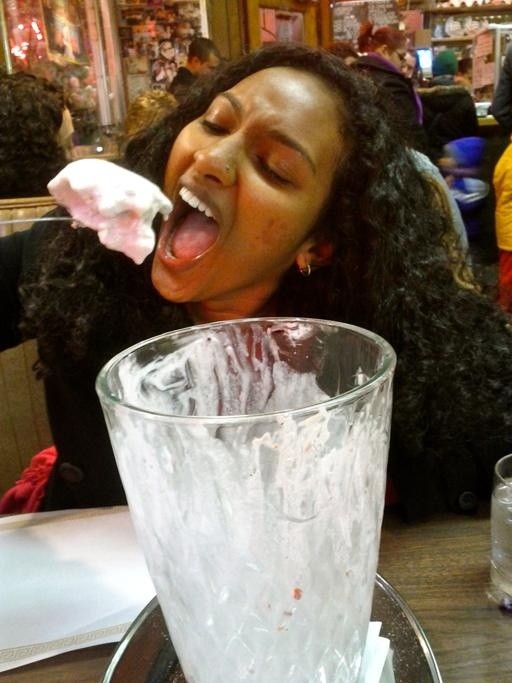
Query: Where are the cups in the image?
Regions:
[484,449,512,608]
[89,314,404,683]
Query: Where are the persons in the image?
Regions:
[1,40,223,199]
[0,39,512,521]
[327,23,511,314]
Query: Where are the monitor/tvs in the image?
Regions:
[416,47,433,79]
